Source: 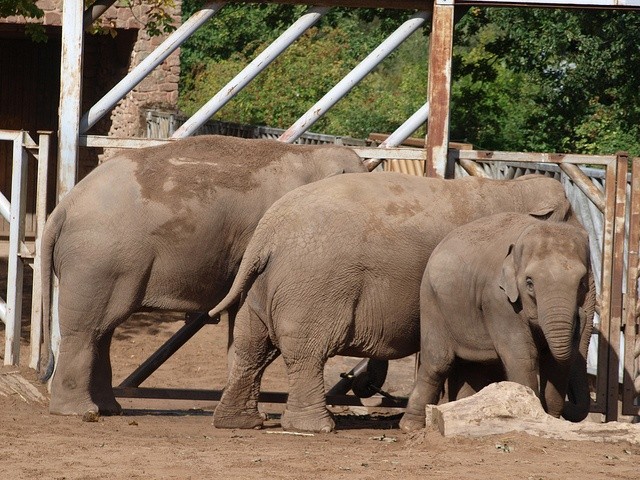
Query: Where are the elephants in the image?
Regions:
[208,174,595,433]
[39,134,388,416]
[399,212,595,432]
